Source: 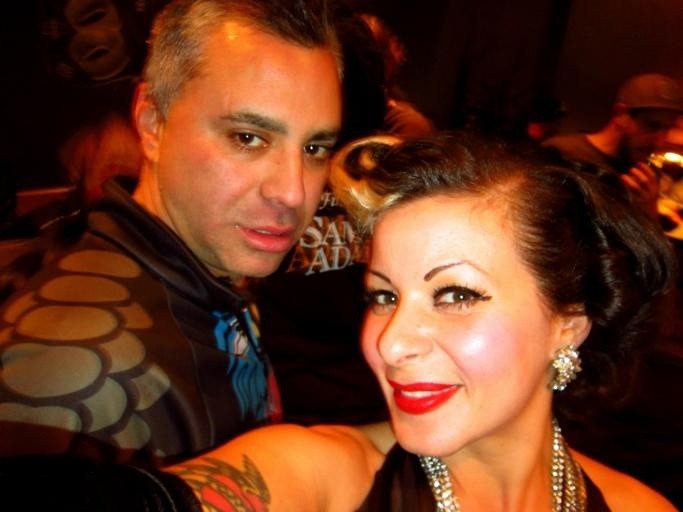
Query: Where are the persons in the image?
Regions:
[0,0,428,468]
[1,128,681,511]
[544,75,682,237]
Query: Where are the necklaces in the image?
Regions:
[414,418,585,512]
